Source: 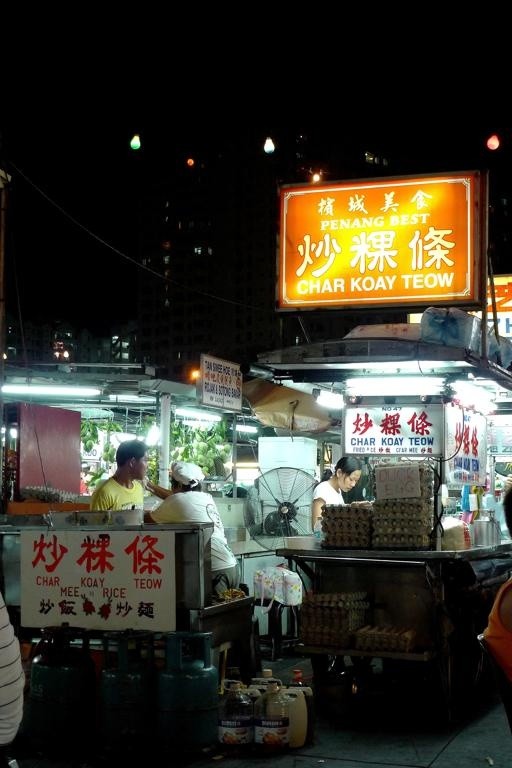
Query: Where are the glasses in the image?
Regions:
[169,471,174,483]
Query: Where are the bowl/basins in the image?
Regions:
[49,509,142,526]
[201,480,225,492]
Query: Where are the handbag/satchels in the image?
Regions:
[254,566,303,614]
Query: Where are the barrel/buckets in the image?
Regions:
[217,666,315,754]
[473,508,509,547]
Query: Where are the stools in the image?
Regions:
[253,597,299,661]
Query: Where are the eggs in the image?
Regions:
[322,497,422,544]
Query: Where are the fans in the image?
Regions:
[241,467,322,646]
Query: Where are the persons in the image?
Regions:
[90,439,151,513]
[143,462,240,603]
[0,590,27,768]
[311,455,362,535]
[481,484,511,687]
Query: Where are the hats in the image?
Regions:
[171,461,205,487]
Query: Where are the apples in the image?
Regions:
[79,418,231,476]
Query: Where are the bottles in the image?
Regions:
[313,516,322,549]
[455,495,461,512]
